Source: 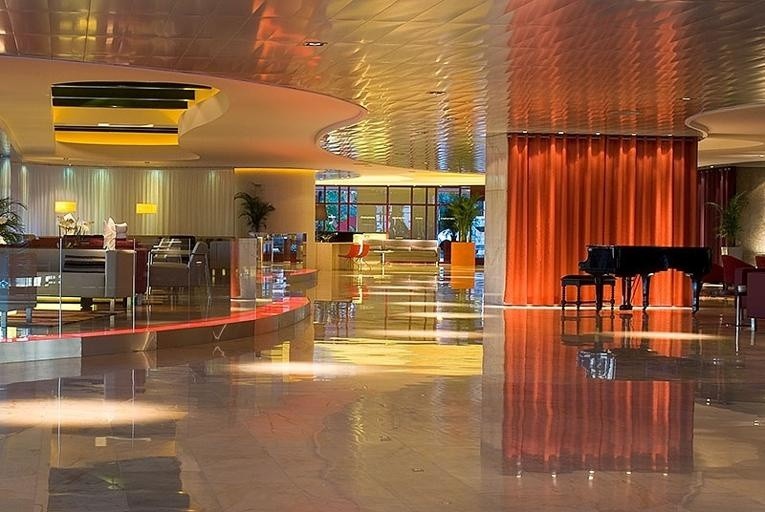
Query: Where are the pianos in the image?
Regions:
[578,245,713,318]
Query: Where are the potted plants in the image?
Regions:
[446,192,484,267]
[708,189,749,260]
[450,267,476,326]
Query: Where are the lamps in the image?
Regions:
[135,202,157,215]
[54,201,77,213]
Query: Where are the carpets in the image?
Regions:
[6,309,117,328]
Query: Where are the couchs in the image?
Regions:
[1,247,137,312]
[366,239,438,264]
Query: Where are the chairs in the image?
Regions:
[338,244,372,273]
[147,241,209,303]
[702,254,765,332]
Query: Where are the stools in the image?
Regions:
[561,274,616,311]
[560,311,615,343]
